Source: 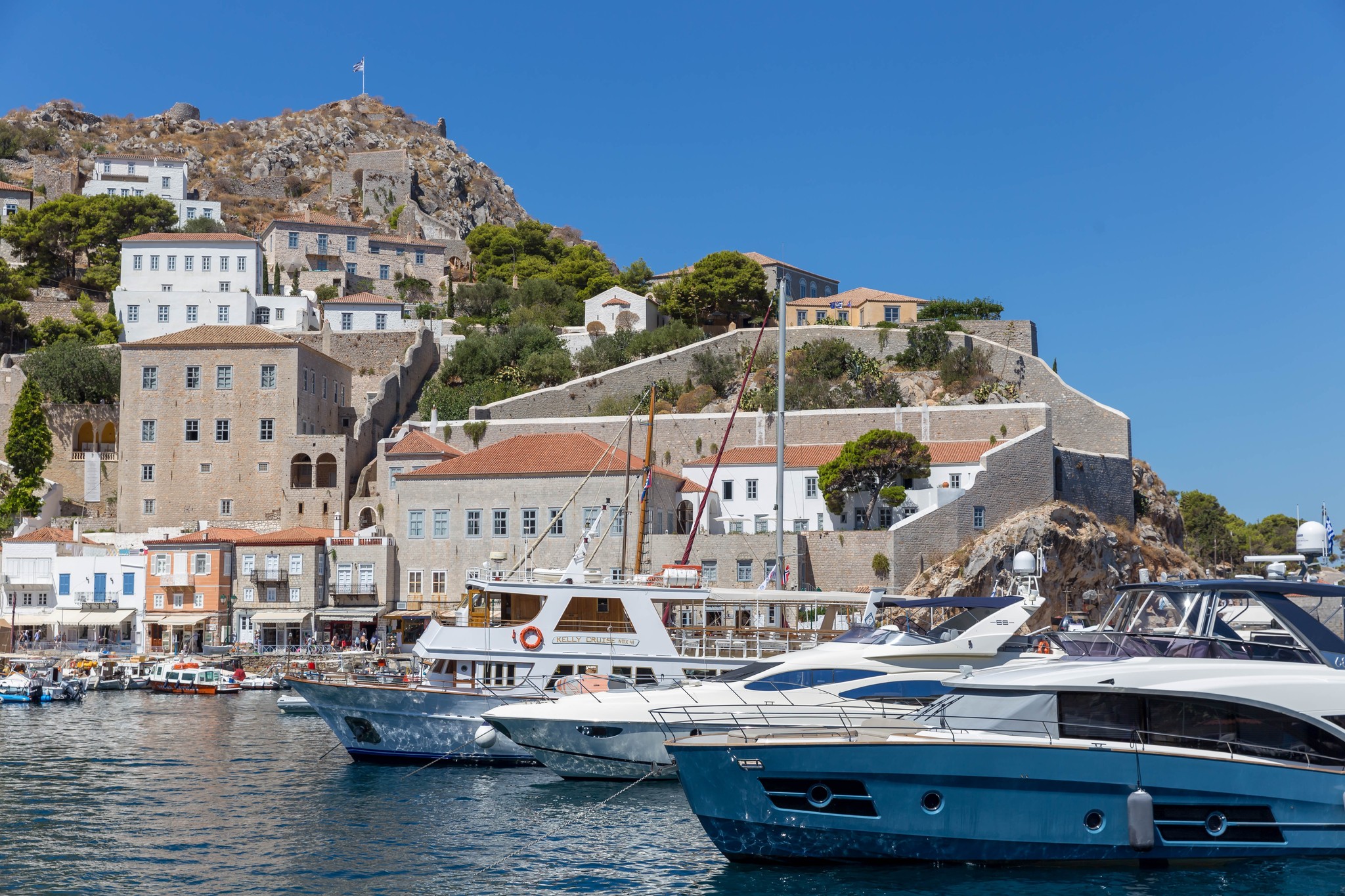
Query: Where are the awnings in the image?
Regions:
[142,616,166,624]
[315,606,386,622]
[250,611,309,623]
[157,616,209,626]
[3,609,137,626]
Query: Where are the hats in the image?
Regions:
[39,630,41,632]
[307,635,310,637]
[63,632,65,633]
[363,633,366,635]
[312,636,315,639]
[335,633,338,635]
[386,633,398,648]
[25,630,29,634]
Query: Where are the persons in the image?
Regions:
[330,632,381,654]
[54,631,67,650]
[83,648,90,652]
[122,632,128,640]
[33,630,43,650]
[304,635,317,654]
[17,630,29,651]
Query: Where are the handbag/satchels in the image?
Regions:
[331,641,334,646]
[54,635,58,640]
[337,639,339,645]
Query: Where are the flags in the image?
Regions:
[27,671,37,687]
[1325,517,1335,554]
[1042,554,1048,573]
[757,564,777,590]
[641,469,652,503]
[781,564,790,586]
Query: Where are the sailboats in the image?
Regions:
[271,279,933,772]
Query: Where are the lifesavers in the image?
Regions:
[1038,640,1050,654]
[519,626,543,649]
[70,662,76,667]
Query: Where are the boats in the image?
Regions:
[475,516,1345,875]
[1,651,429,718]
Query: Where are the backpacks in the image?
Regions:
[313,639,317,645]
[25,635,27,640]
[21,637,25,642]
